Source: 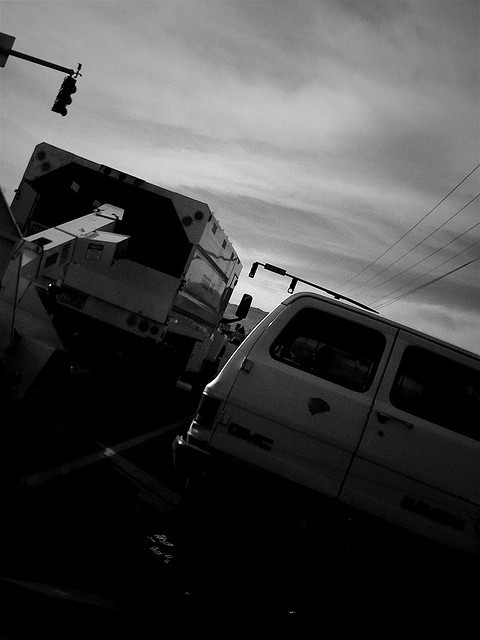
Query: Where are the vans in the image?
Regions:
[170,290,479,556]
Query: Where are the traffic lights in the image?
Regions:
[51,76,77,118]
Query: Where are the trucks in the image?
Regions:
[1,143,254,377]
